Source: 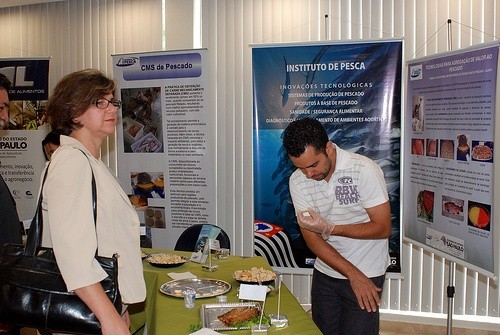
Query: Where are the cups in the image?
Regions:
[217,248,229,260]
[183,288,195,311]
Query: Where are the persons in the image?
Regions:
[1,74,23,335]
[282,118,394,335]
[42,129,63,160]
[36,69,147,335]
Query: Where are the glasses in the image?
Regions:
[89,98,122,110]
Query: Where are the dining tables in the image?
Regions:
[140,247,324,335]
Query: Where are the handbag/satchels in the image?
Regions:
[0,147,123,335]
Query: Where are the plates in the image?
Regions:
[144,256,187,268]
[142,252,146,260]
[233,268,277,286]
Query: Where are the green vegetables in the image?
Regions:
[234,315,271,330]
